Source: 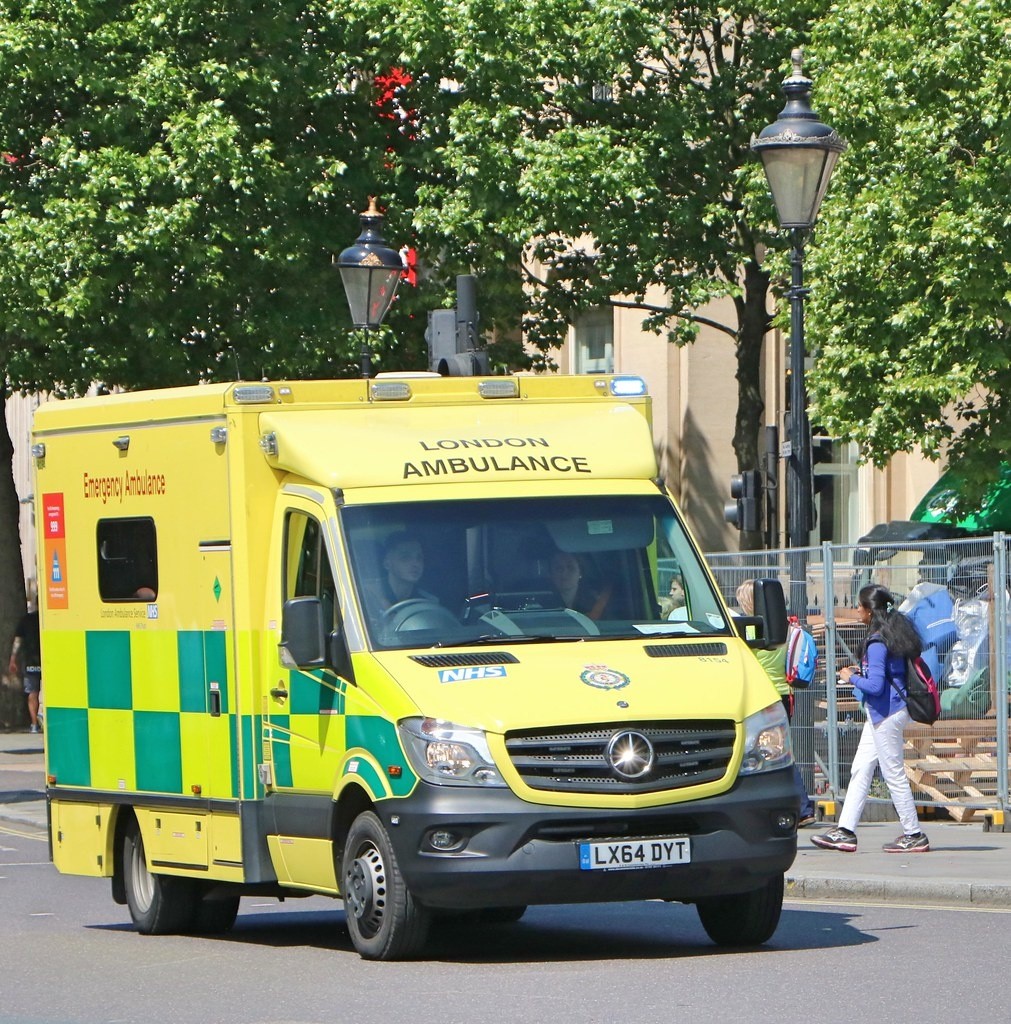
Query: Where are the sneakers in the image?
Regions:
[811,827,856,851]
[882,834,930,851]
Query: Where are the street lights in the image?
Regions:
[334,196,405,381]
[755,73,848,823]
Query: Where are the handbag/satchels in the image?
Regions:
[905,590,958,688]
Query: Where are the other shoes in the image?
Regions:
[798,812,815,827]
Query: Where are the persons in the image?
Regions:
[539,547,618,620]
[668,574,688,621]
[361,531,440,625]
[98,523,156,599]
[736,579,816,828]
[8,594,44,733]
[810,584,930,853]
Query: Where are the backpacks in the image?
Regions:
[783,616,818,686]
[867,633,942,726]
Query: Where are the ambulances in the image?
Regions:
[32,370,804,965]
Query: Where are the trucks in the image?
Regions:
[727,471,758,535]
[851,452,1011,610]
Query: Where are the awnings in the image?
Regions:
[910,451,1011,533]
